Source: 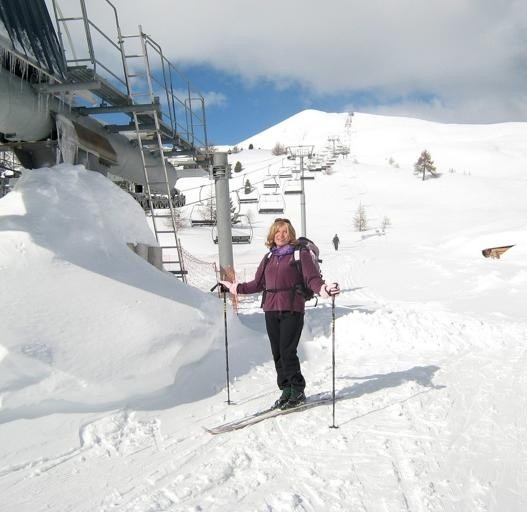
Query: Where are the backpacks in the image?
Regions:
[263,237,322,301]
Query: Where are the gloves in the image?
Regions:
[319,282,341,298]
[218,281,241,295]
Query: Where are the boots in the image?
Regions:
[272,384,306,410]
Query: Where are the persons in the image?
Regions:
[333,234,339,250]
[219,218,340,411]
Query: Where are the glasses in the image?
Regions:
[275,218,291,224]
